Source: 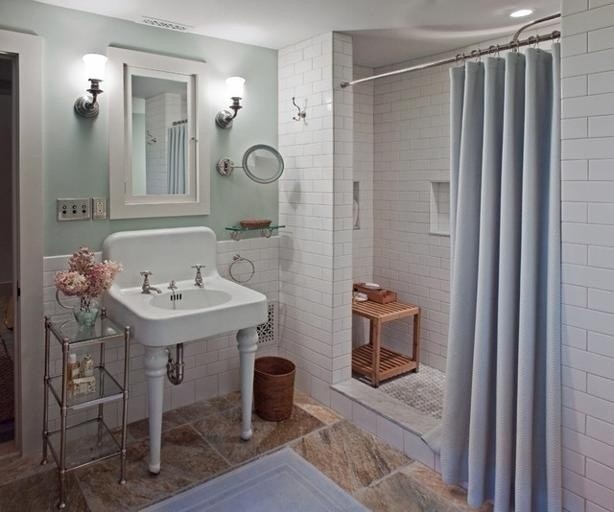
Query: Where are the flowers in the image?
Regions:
[53,246,123,309]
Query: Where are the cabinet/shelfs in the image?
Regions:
[41,313,131,511]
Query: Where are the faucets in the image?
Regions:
[190,264,206,288]
[140,270,162,295]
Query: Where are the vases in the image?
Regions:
[74,309,99,328]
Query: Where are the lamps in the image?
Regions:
[74,52,108,119]
[215,75,245,128]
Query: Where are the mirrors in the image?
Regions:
[216,142,284,183]
[107,46,211,220]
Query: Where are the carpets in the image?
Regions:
[132,448,373,512]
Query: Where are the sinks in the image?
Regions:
[102,225,269,347]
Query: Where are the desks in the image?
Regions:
[352,299,421,389]
